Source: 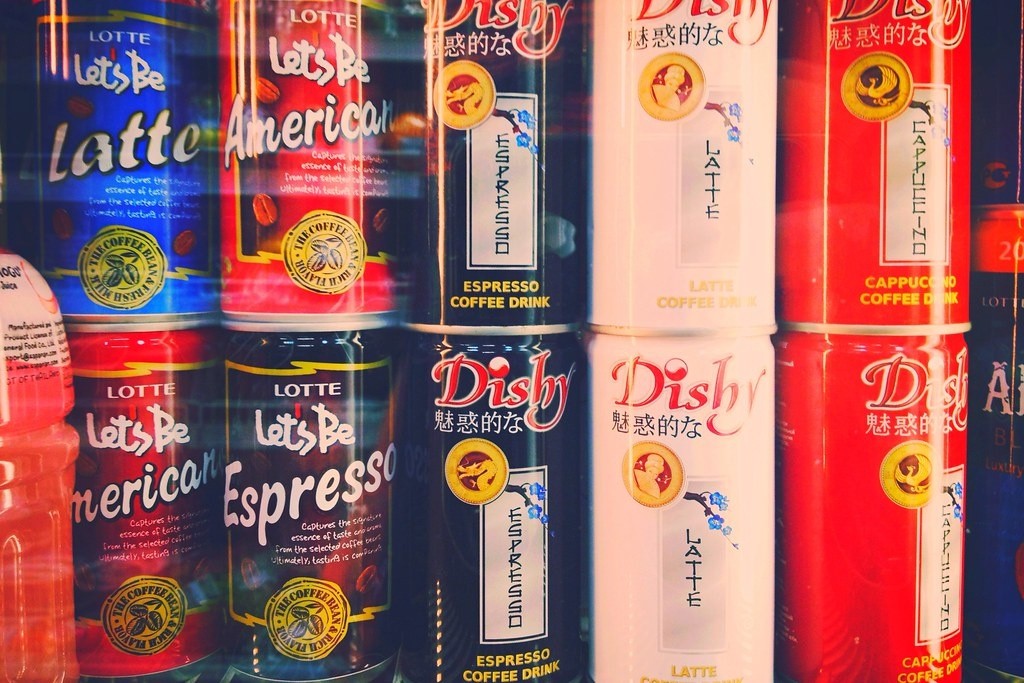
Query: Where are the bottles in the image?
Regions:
[0,245,81,682]
